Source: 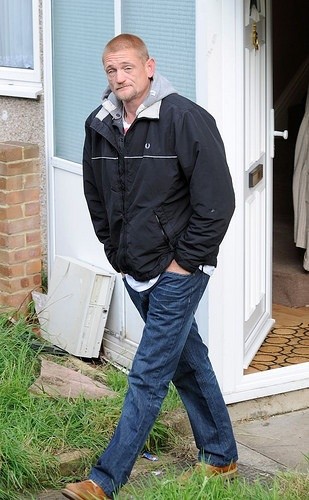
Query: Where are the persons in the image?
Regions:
[59,34,239,500]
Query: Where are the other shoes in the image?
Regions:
[178,462,239,485]
[61,479,113,500]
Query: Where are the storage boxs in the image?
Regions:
[40,254,118,358]
